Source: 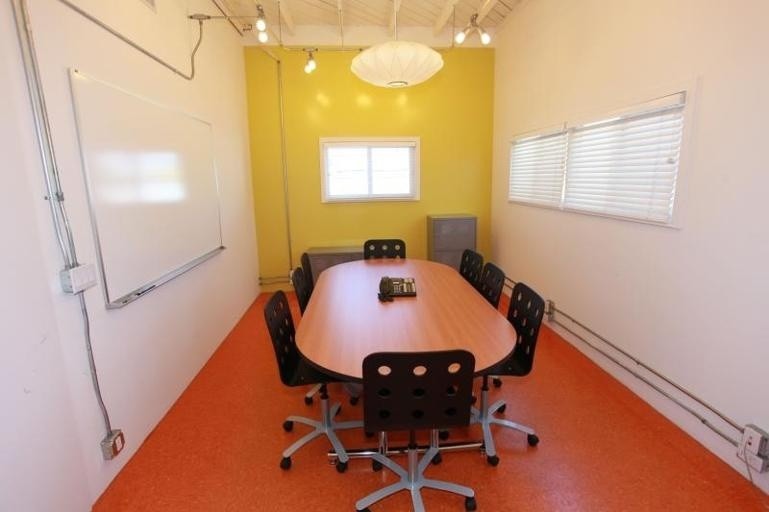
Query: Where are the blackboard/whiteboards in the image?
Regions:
[69,67,227,310]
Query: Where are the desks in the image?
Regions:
[294,258,520,461]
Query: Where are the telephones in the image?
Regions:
[381,277,416,296]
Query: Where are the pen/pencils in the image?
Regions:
[135,285,156,295]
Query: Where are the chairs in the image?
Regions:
[300,252,315,294]
[458,248,484,289]
[363,238,407,260]
[261,289,366,474]
[474,261,506,309]
[438,280,544,466]
[352,346,480,512]
[290,266,311,314]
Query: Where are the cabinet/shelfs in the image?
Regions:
[424,210,477,270]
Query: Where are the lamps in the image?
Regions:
[186,4,272,48]
[350,0,492,96]
